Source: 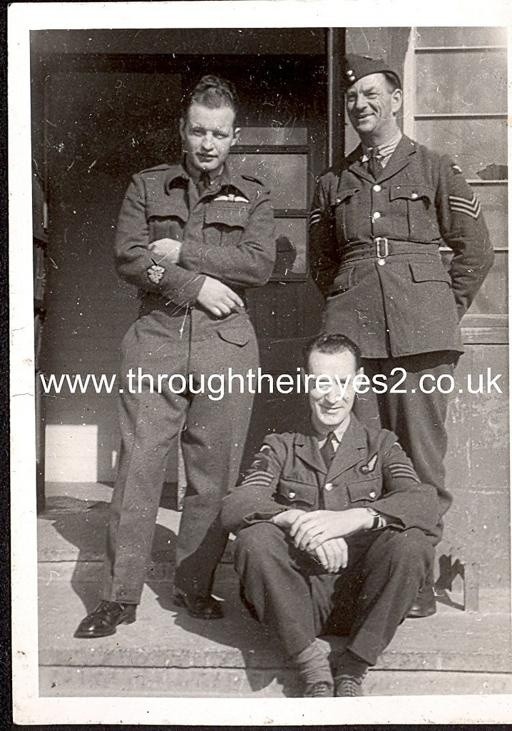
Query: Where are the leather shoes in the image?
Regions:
[405,581,437,621]
[302,672,367,697]
[172,580,224,622]
[73,598,138,641]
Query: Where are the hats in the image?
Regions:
[338,54,401,90]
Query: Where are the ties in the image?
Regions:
[367,148,384,178]
[320,432,336,466]
[195,172,210,196]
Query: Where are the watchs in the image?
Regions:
[365,502,380,530]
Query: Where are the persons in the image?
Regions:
[218,333,442,697]
[78,76,276,639]
[307,54,494,616]
